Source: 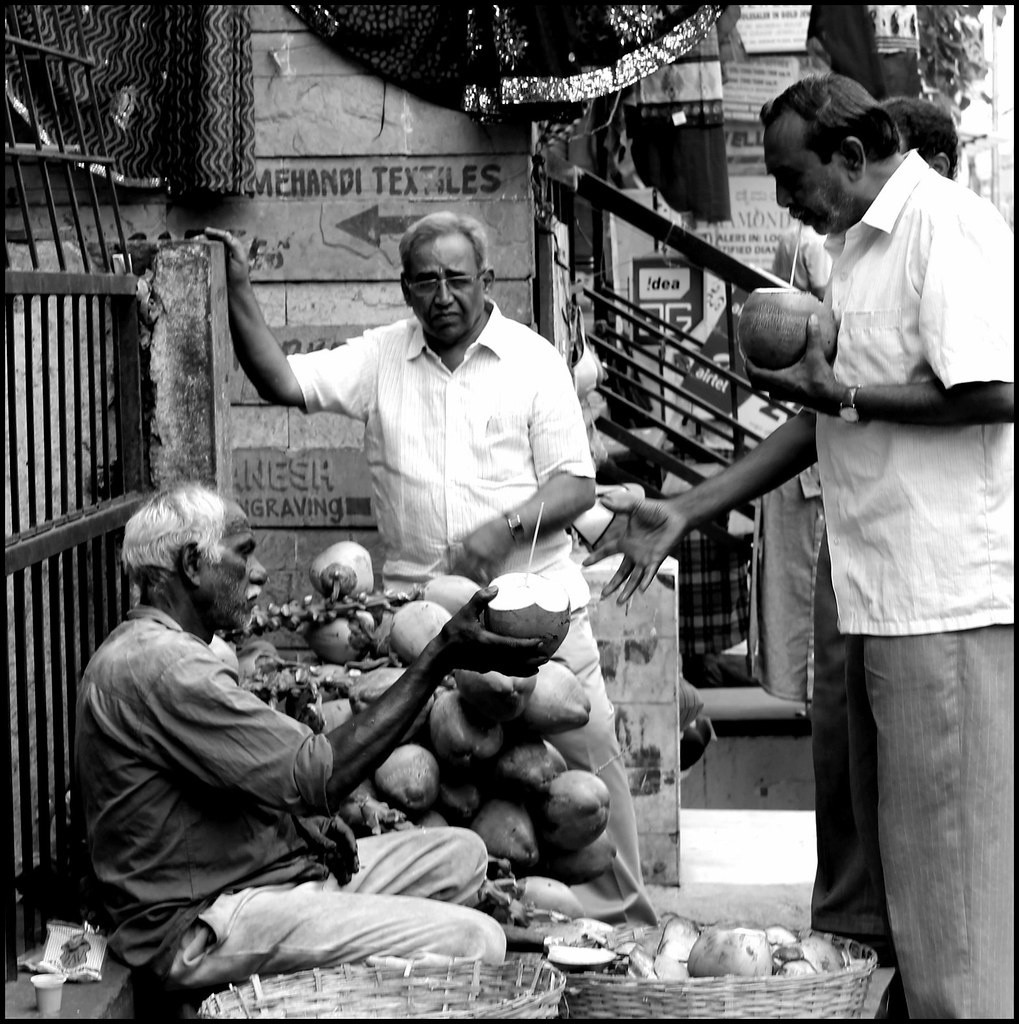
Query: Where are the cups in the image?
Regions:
[31,973,66,1013]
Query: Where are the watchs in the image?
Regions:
[838,386,870,424]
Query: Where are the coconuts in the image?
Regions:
[240,541,848,981]
[737,287,838,370]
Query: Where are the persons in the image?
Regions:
[582,77,1014,1022]
[187,210,656,925]
[74,480,546,985]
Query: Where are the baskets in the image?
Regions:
[554,920,878,1019]
[196,955,566,1018]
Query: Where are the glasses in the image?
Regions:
[403,268,482,297]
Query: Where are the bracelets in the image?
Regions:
[504,507,524,545]
